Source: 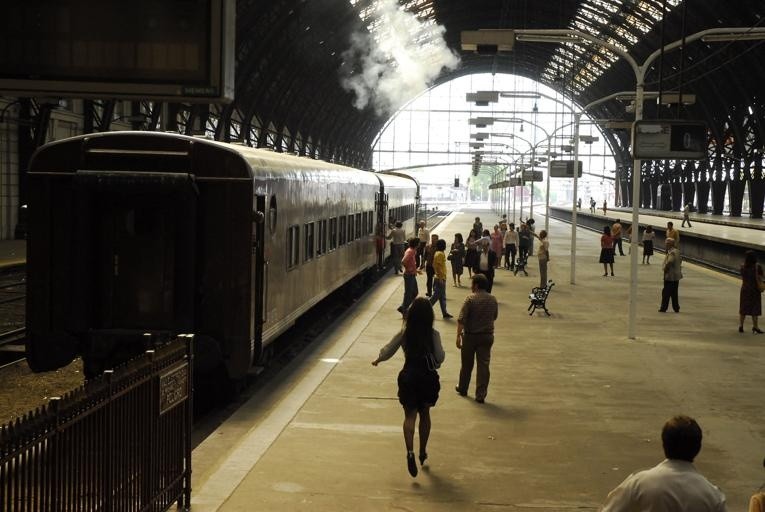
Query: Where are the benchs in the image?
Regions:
[528,279,555,316]
[514,253,528,276]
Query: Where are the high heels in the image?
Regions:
[752,327,765,334]
[419,453,427,465]
[407,452,417,477]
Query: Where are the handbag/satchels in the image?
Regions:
[424,327,440,372]
[755,262,765,292]
[447,251,456,261]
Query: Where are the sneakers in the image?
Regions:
[442,313,452,318]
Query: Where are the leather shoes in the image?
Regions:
[456,384,467,396]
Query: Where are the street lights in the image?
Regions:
[460,113,631,260]
[466,84,700,286]
[452,23,761,342]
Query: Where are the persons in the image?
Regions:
[682,202,692,227]
[590,197,595,213]
[375,214,550,317]
[598,415,727,512]
[603,200,607,215]
[371,298,445,478]
[738,251,764,333]
[578,198,582,211]
[599,218,683,313]
[455,274,498,403]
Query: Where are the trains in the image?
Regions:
[25,129,420,391]
